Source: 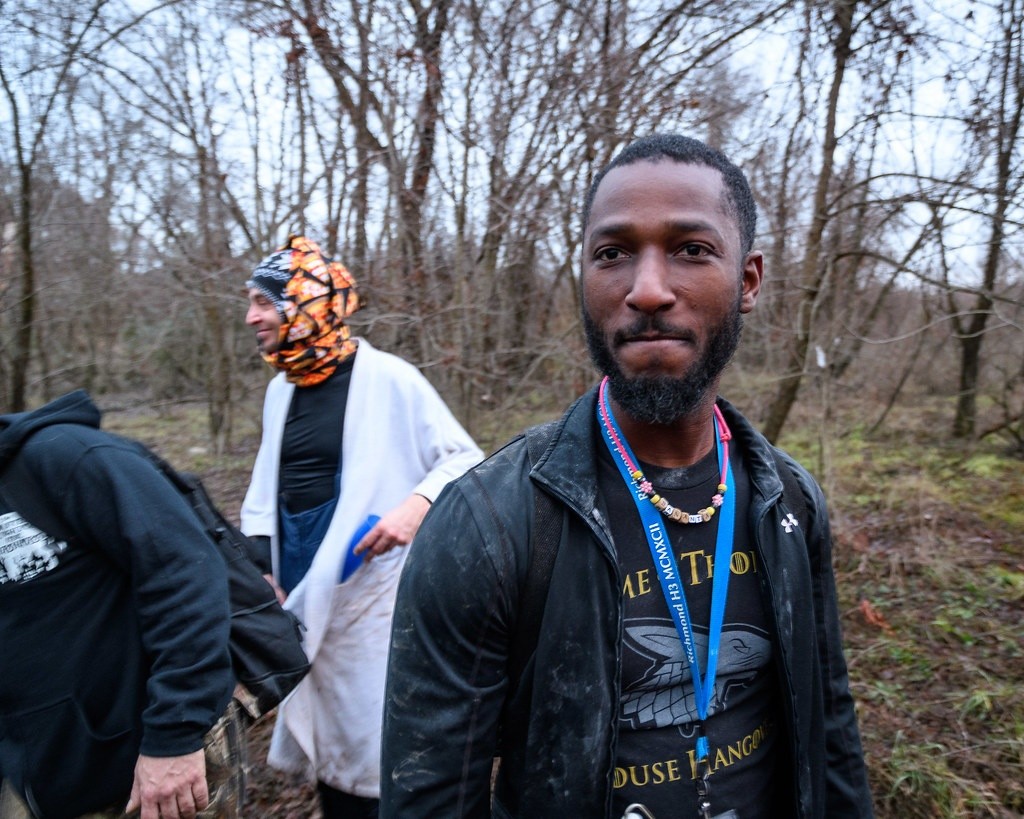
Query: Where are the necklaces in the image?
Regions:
[599,376,732,524]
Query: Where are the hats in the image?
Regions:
[244,249,291,325]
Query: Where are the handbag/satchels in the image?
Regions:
[125,437,311,718]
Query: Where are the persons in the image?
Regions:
[0,387,234,819]
[379,133,874,819]
[238,232,486,819]
